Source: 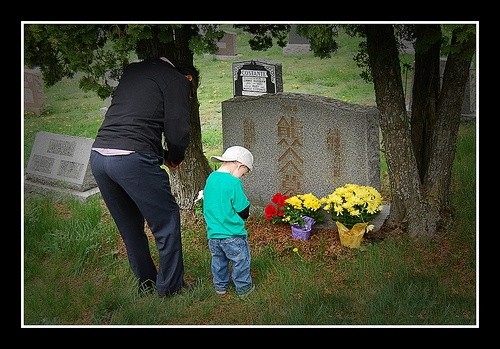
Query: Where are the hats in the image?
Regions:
[211,145,254,171]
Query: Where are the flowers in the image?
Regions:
[284,193,325,228]
[265,193,305,227]
[323,183,384,233]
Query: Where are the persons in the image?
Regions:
[202,146,256,299]
[92,56,201,297]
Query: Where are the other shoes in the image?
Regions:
[138,280,157,297]
[158,283,189,297]
[238,283,256,299]
[215,290,226,295]
